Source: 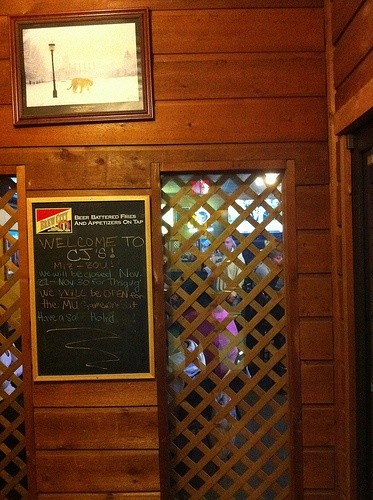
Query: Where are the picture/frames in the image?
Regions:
[7,7,155,127]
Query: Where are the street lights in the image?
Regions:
[48,44,58,98]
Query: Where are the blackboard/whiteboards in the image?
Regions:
[23,195,155,382]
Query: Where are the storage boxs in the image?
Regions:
[167,236,200,271]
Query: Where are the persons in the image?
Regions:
[0,315,28,500]
[177,268,240,462]
[203,236,251,379]
[182,197,284,235]
[247,233,287,396]
